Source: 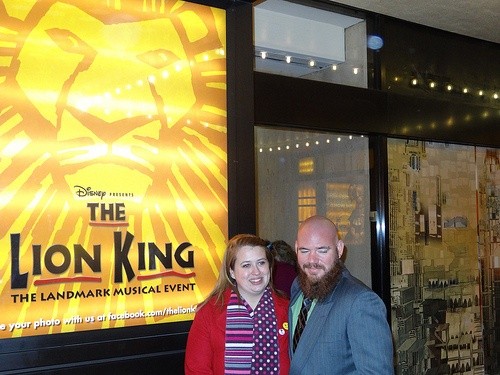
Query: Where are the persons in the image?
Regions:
[268,240,299,299]
[184,233,290,375]
[289,216,395,375]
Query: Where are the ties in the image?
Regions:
[293,297,315,353]
